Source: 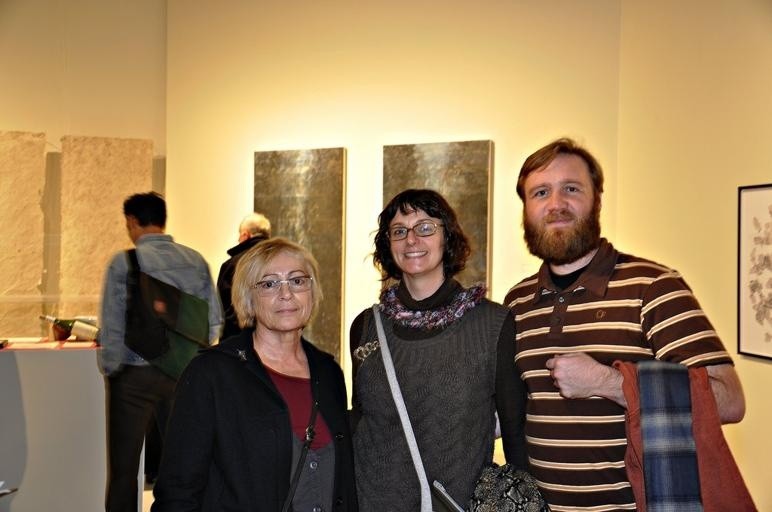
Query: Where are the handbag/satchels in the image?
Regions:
[124,268,210,383]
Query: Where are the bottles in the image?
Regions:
[45,315,99,342]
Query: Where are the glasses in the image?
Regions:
[248,275,313,298]
[386,220,444,242]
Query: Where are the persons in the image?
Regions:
[149,237,359,511]
[494,136,747,512]
[97,191,224,512]
[348,189,532,512]
[216,213,273,342]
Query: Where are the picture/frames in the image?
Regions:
[738,183,772,361]
